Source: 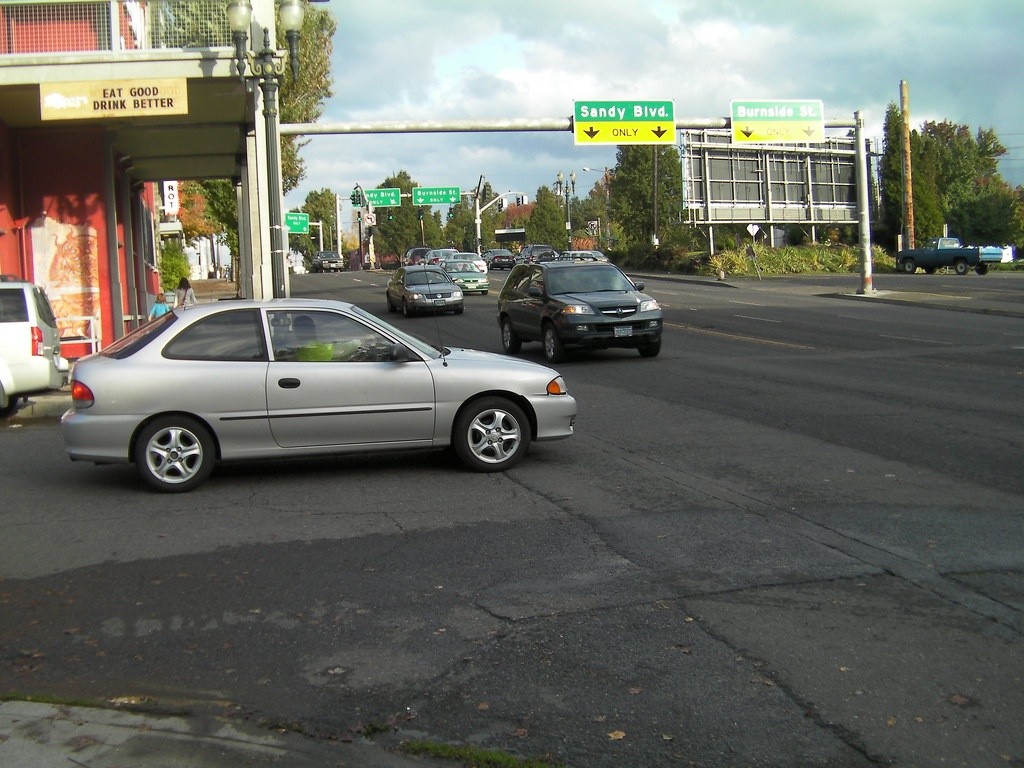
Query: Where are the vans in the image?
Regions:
[0,280,71,422]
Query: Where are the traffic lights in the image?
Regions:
[388,216,394,220]
[357,217,361,223]
[350,193,362,204]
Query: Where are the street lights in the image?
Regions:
[557,171,577,251]
[583,165,611,250]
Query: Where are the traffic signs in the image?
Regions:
[572,99,678,146]
[285,212,310,235]
[351,188,401,207]
[730,97,825,145]
[413,186,461,205]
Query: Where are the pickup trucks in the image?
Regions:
[893,236,1003,276]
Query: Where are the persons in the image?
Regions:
[293,316,376,362]
[148,293,170,321]
[209,263,216,278]
[173,278,197,309]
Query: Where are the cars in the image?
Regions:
[481,248,514,270]
[402,246,489,296]
[512,243,612,265]
[60,295,579,495]
[385,265,464,318]
[313,249,344,272]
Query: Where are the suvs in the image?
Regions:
[495,257,666,365]
